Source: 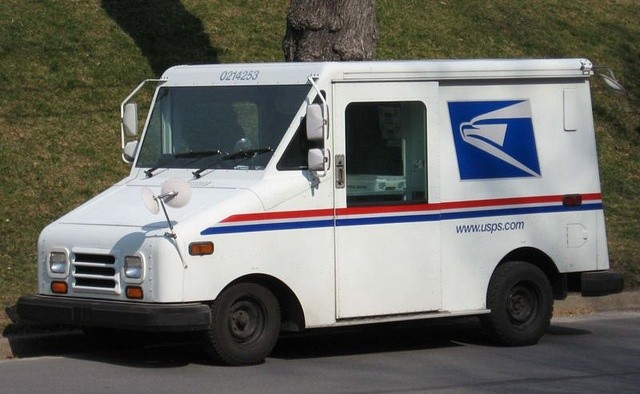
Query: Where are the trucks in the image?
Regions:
[17,57,623,365]
